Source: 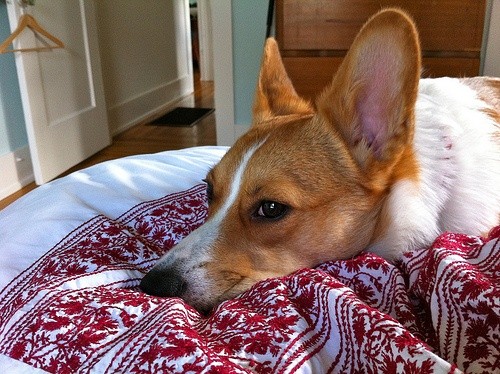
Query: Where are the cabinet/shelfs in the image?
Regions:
[275,0,487,113]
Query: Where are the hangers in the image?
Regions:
[0,0,65,54]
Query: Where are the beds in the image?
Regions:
[0,145,500,374]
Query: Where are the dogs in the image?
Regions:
[137,7,500,324]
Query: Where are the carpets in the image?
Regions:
[144,106,216,128]
